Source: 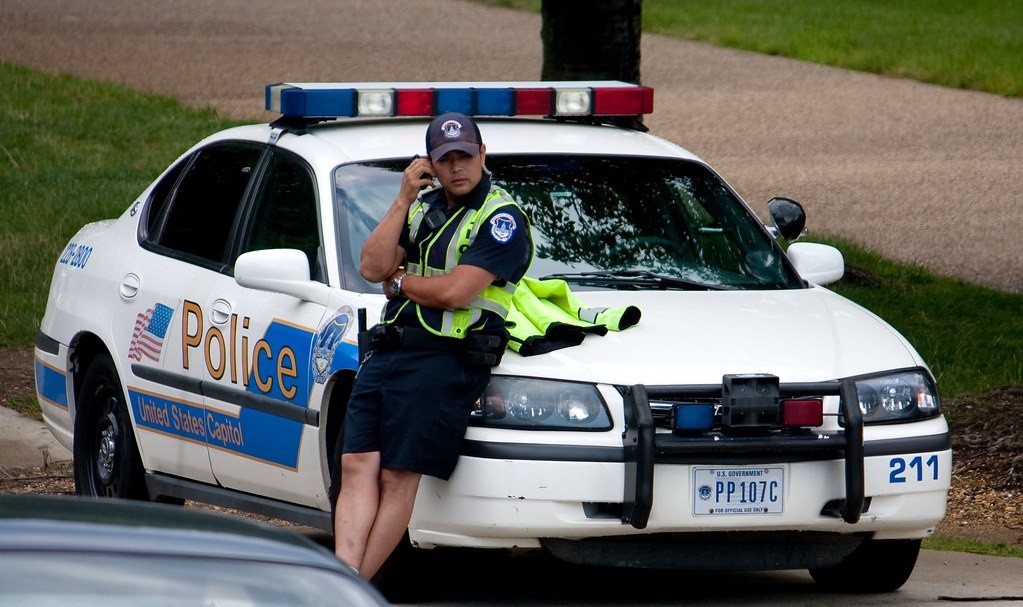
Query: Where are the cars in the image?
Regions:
[0,493,389,607]
[34,81,952,607]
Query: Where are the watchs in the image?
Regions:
[389,272,409,297]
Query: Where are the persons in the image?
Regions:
[334,111,536,582]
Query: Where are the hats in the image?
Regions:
[425,112,481,162]
